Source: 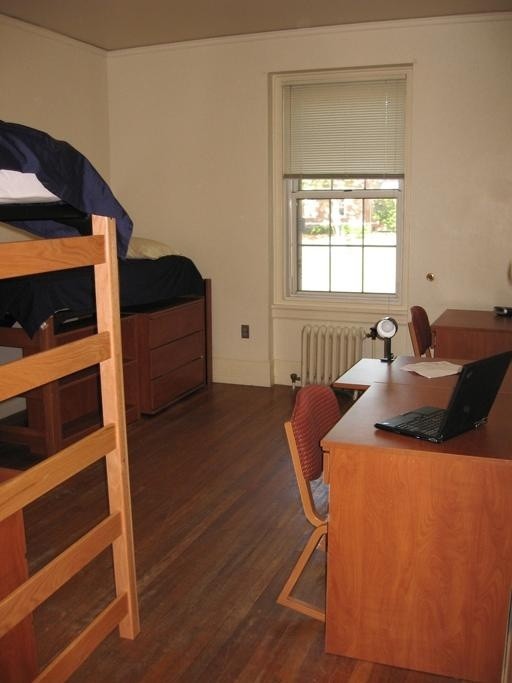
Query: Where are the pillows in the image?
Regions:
[126,236,186,259]
[0,166,64,205]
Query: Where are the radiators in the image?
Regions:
[300,323,368,399]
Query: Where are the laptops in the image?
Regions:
[374,350,512,444]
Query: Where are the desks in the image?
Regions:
[1,465,41,683]
[332,357,511,401]
[432,307,512,362]
[318,382,511,683]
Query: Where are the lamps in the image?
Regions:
[370,316,398,362]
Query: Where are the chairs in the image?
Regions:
[275,383,341,623]
[408,305,433,358]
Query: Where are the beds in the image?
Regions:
[1,203,145,682]
[0,275,212,460]
